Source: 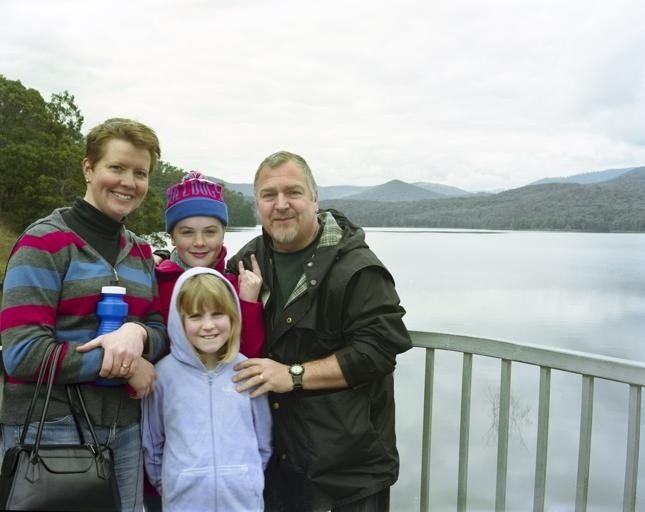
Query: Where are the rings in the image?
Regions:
[122,363,129,369]
[260,374,264,383]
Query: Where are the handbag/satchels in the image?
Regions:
[3,341,123,512]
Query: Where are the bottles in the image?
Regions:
[93,285,128,386]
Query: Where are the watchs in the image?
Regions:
[289,362,305,392]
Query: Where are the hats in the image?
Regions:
[165,172,228,235]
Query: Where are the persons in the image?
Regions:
[0,118,168,510]
[226,150,412,512]
[150,169,265,359]
[139,265,274,512]
